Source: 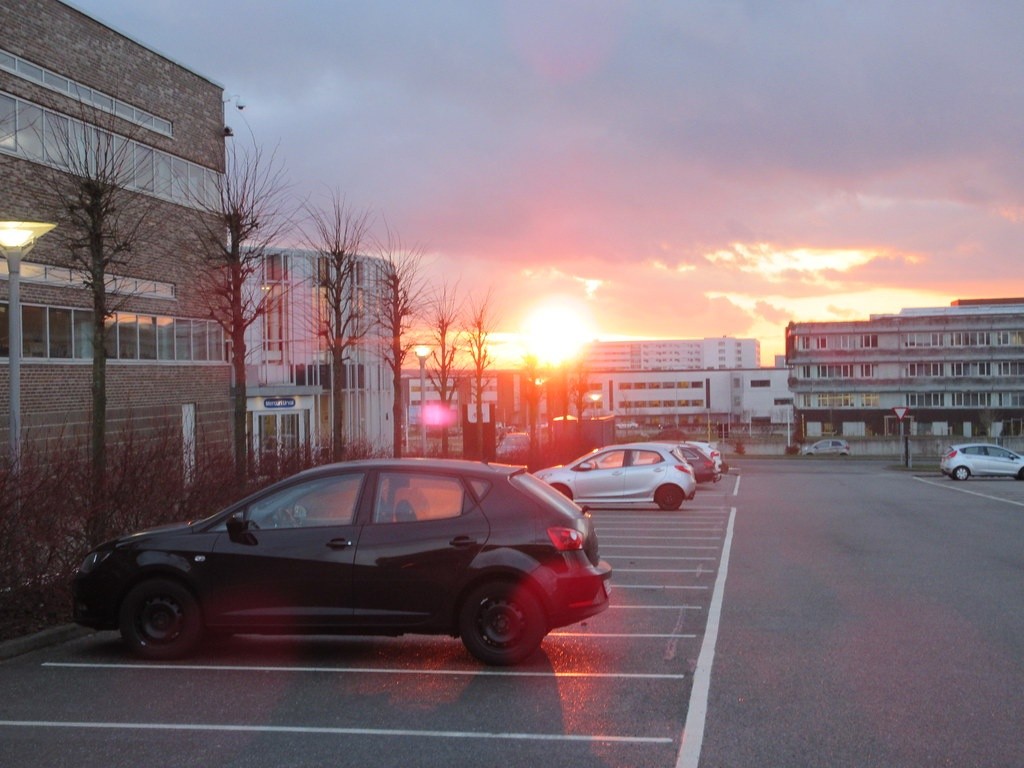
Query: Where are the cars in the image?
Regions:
[596,440,729,484]
[71,454,617,669]
[940,442,1024,481]
[531,443,697,511]
[804,439,851,456]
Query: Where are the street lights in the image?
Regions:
[0,218,60,511]
[588,389,604,418]
[413,344,431,459]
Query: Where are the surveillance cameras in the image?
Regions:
[236,99,246,110]
[224,126,232,134]
